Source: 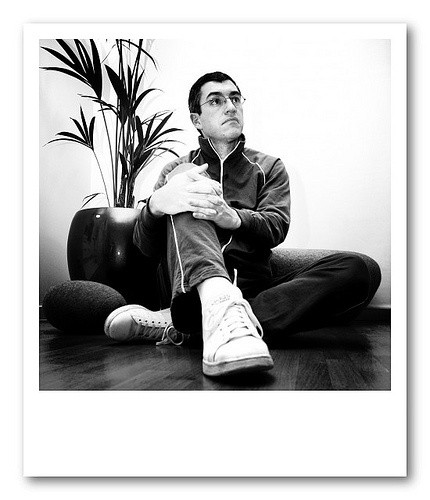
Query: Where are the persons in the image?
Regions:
[104,71,382,377]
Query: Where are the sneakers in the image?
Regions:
[104,304,191,346]
[203,268,274,376]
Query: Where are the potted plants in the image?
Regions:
[39,39,187,312]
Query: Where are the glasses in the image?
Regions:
[198,96,246,108]
